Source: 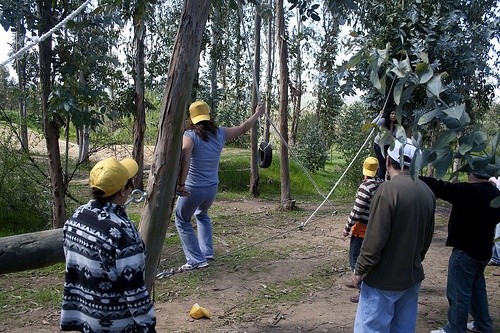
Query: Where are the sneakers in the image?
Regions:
[492,268,500,276]
[181,262,208,269]
[345,279,353,286]
[488,261,495,265]
[350,293,360,302]
[430,330,447,333]
[204,255,213,258]
[467,321,479,331]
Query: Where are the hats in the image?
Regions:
[363,157,379,177]
[190,303,210,319]
[89,159,138,197]
[189,101,210,124]
[387,138,422,166]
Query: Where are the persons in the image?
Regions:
[488,176,500,266]
[59,157,156,333]
[367,109,399,183]
[343,157,384,302]
[418,173,500,333]
[352,138,436,333]
[174,101,266,271]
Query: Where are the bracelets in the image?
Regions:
[353,269,362,276]
[178,183,186,187]
